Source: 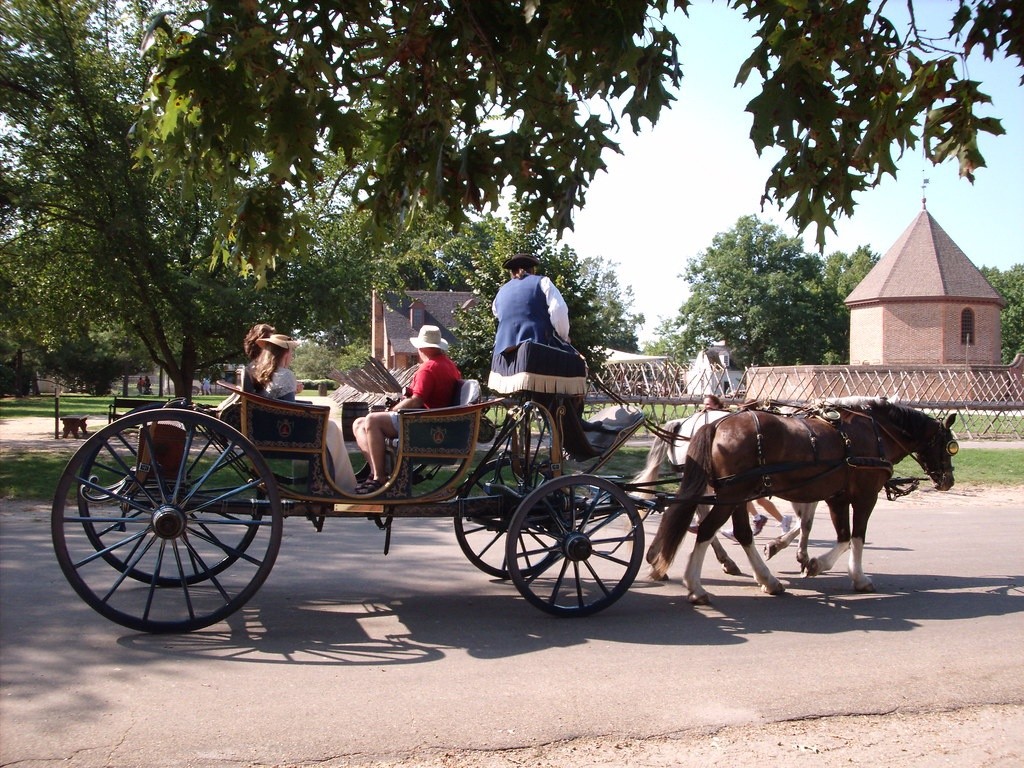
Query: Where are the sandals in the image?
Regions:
[355,474,385,495]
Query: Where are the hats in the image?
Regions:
[502,253,539,269]
[256,334,297,350]
[410,324,449,350]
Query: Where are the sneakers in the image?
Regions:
[688,525,699,533]
[721,529,739,542]
[777,515,792,533]
[753,515,769,536]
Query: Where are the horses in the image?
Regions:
[650,400,957,605]
[627,395,904,573]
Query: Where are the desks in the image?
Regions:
[60,414,89,438]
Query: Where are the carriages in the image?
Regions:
[50,338,961,634]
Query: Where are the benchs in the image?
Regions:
[217,364,330,461]
[370,378,506,466]
[109,397,170,428]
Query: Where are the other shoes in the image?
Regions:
[581,418,604,432]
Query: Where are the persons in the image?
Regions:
[243,323,358,494]
[137,378,143,393]
[491,252,603,434]
[145,376,151,390]
[687,395,792,542]
[351,324,460,494]
[200,378,212,395]
